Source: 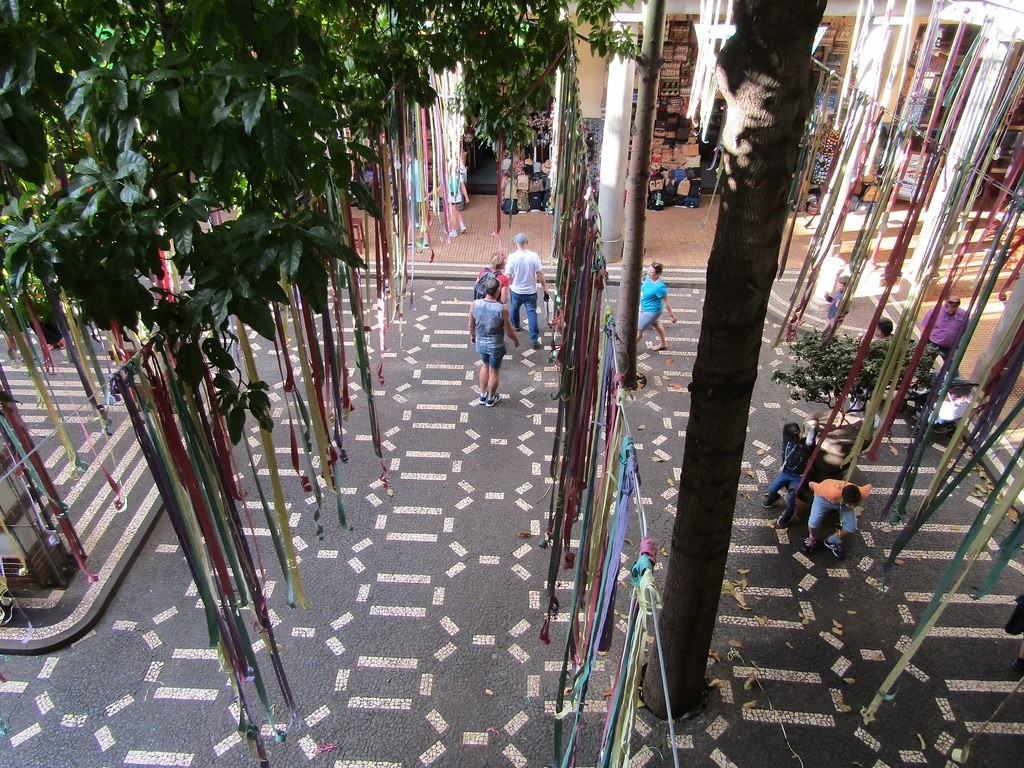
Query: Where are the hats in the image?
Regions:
[542,160,552,174]
[515,233,527,244]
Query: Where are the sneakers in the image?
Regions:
[824,536,842,557]
[479,390,489,404]
[485,393,499,406]
[804,537,818,551]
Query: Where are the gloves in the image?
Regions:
[543,291,549,302]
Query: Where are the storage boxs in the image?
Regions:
[678,144,700,169]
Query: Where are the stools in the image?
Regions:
[352,218,365,255]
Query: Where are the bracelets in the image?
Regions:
[670,314,673,317]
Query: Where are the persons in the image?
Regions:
[850,318,894,426]
[802,479,872,559]
[476,251,510,305]
[406,146,470,248]
[506,233,550,349]
[469,278,520,406]
[761,419,817,527]
[919,295,968,380]
[637,262,678,351]
[822,276,850,335]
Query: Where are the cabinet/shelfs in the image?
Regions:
[660,16,852,137]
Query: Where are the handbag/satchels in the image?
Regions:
[806,116,885,215]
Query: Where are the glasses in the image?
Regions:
[652,261,660,270]
[946,300,960,306]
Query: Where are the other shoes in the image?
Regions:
[777,509,792,527]
[762,493,781,509]
[1012,661,1024,672]
[531,340,539,348]
[652,345,667,351]
[511,324,520,331]
[407,225,466,249]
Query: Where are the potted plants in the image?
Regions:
[768,325,945,477]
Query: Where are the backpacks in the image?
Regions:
[647,110,700,211]
[501,156,548,214]
[473,268,501,301]
